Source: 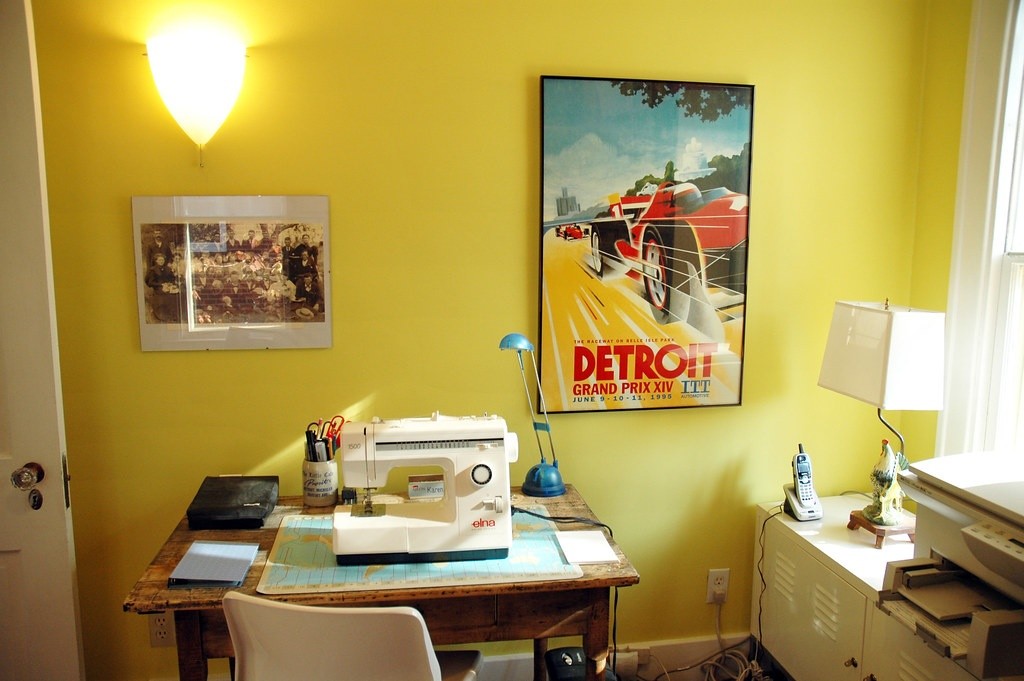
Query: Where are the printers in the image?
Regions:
[875,449,1024,681]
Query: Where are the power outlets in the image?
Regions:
[706,568,730,604]
[148,612,176,648]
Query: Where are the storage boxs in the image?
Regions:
[186,475,280,530]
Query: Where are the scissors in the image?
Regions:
[326,415,352,438]
[307,421,331,438]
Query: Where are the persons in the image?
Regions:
[146,222,324,322]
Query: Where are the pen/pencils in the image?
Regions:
[305,418,336,462]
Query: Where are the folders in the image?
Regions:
[168,538,260,588]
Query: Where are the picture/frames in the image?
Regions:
[536,75,756,415]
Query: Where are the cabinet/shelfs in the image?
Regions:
[749,493,981,681]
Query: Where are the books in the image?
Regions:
[167,539,259,587]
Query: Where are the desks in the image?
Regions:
[123,484,640,680]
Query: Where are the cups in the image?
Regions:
[302,459,338,506]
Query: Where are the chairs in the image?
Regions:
[222,592,483,680]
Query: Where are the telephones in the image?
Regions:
[784,445,823,522]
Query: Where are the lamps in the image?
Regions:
[499,334,563,497]
[818,299,945,523]
